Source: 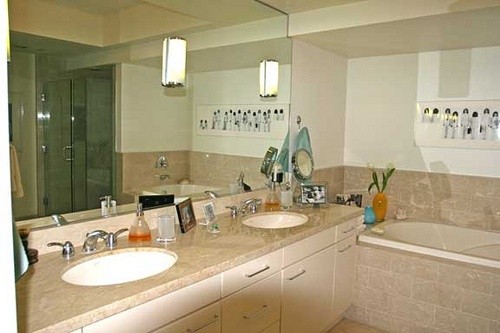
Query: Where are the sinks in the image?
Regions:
[243,212,309,229]
[60,248,178,287]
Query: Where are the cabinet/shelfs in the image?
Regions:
[333,213,368,329]
[281,226,337,332]
[82,274,221,332]
[221,249,282,333]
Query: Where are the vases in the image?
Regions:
[372,193,387,221]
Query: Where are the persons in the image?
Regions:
[423,106,430,120]
[471,112,480,140]
[460,109,469,138]
[449,111,458,138]
[480,108,492,140]
[493,111,499,139]
[199,108,285,132]
[442,108,451,138]
[433,107,441,122]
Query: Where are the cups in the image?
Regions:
[156,216,176,242]
[229,183,238,193]
[281,190,293,209]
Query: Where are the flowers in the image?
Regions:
[366,161,396,192]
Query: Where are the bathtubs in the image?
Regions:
[358,218,500,269]
[142,184,218,197]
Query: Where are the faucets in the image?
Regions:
[82,230,109,253]
[51,214,68,225]
[239,199,255,215]
[204,189,216,198]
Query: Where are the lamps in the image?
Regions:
[160,36,187,88]
[259,58,279,97]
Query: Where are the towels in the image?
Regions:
[296,128,313,158]
[13,225,30,282]
[276,131,290,172]
[9,144,24,199]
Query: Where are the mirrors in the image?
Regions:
[6,1,294,221]
[263,145,279,176]
[295,148,313,182]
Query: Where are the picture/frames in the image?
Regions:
[175,198,197,233]
[300,181,327,205]
[412,99,500,150]
